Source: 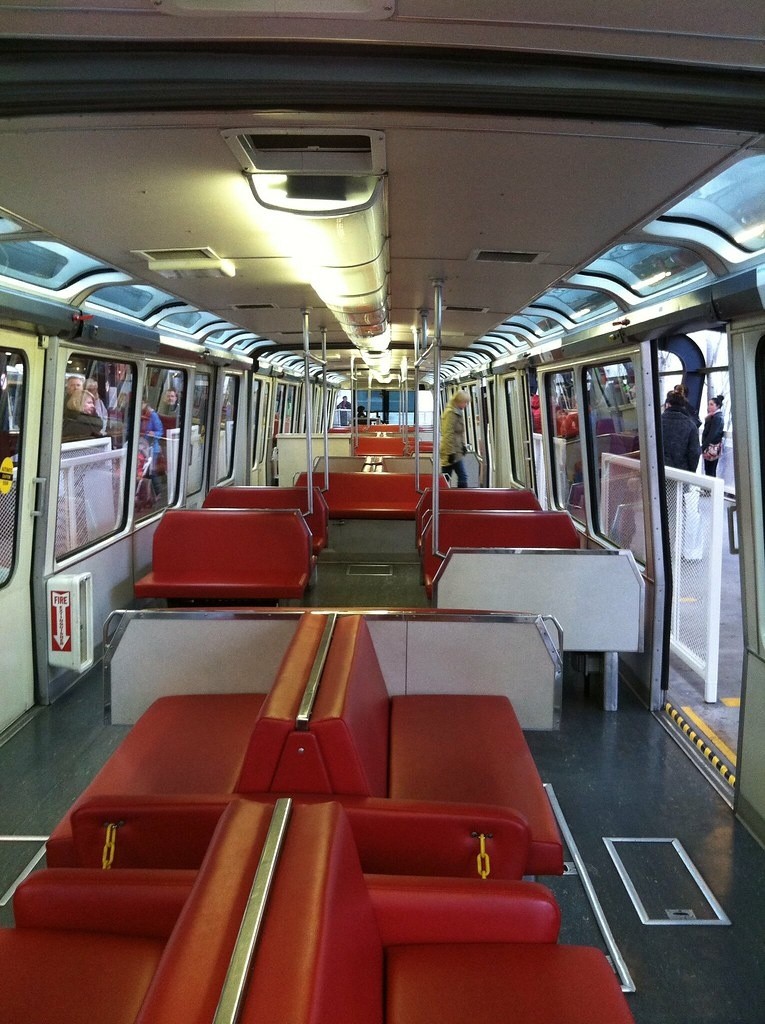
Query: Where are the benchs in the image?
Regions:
[198,485,329,589]
[134,508,312,609]
[292,472,454,521]
[423,510,582,608]
[415,486,544,585]
[327,424,434,456]
[567,418,642,548]
[0,610,636,1023]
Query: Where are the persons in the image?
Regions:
[674,385,702,426]
[553,368,642,513]
[438,390,469,487]
[661,392,701,474]
[700,395,725,481]
[357,406,367,418]
[0,358,232,507]
[336,396,352,409]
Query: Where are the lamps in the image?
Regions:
[241,168,395,383]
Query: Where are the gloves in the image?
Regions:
[461,445,468,457]
[447,453,457,463]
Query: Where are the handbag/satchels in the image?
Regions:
[702,441,723,460]
